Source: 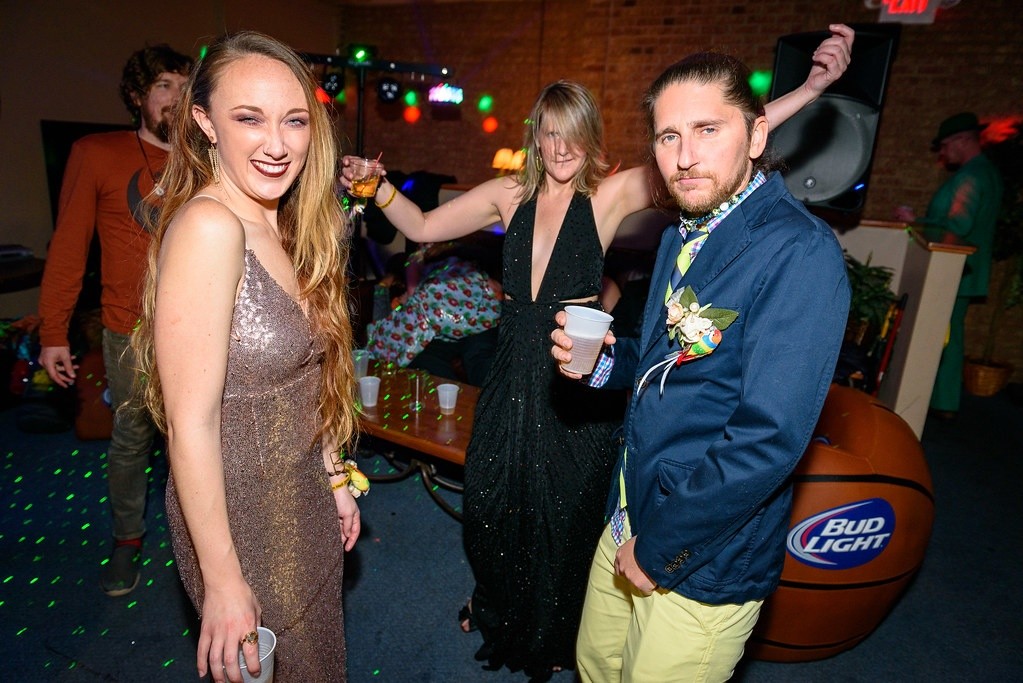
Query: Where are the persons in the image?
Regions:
[114,31,363,683]
[339,23,857,683]
[37,45,207,599]
[895,112,1003,423]
[549,54,851,683]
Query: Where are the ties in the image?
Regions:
[664,223,711,307]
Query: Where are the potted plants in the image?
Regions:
[963,255,1023,397]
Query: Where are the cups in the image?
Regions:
[437,383,459,415]
[349,157,384,198]
[407,368,429,413]
[352,350,369,382]
[561,306,615,375]
[359,376,381,407]
[221,627,276,683]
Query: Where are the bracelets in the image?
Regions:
[327,459,371,498]
[374,186,397,210]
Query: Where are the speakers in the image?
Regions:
[765,22,903,232]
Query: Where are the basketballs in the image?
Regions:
[743,381,937,663]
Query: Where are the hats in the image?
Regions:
[932,111,989,144]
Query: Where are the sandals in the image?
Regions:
[552,665,564,673]
[457,599,478,633]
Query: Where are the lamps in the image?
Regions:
[320,63,348,97]
[374,72,407,101]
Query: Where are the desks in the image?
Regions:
[349,366,482,524]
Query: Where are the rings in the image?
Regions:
[238,630,260,647]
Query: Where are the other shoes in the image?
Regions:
[929,406,955,424]
[100,534,144,596]
